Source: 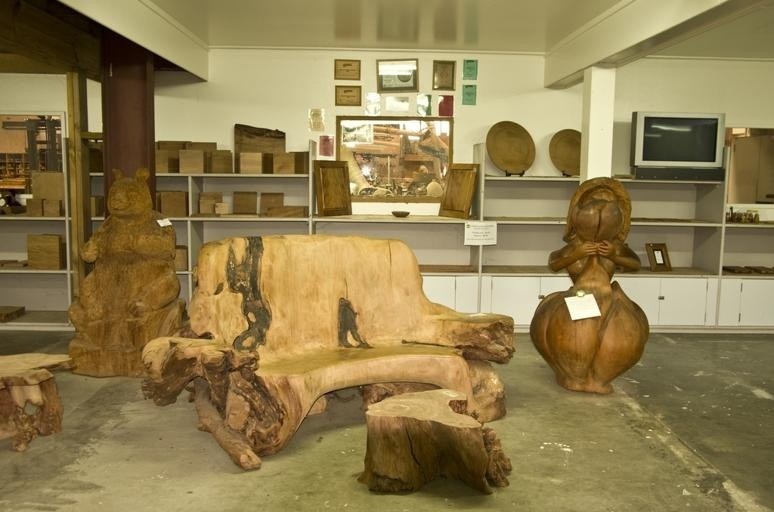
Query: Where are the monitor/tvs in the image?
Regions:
[630,110,726,168]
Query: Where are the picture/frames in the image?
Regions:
[438,164,480,220]
[432,60,457,91]
[375,58,419,94]
[416,95,432,116]
[365,92,380,117]
[234,124,286,174]
[335,115,454,203]
[319,136,334,156]
[463,59,478,81]
[308,107,326,132]
[335,85,362,106]
[384,96,410,112]
[334,59,361,80]
[311,159,353,217]
[439,95,453,116]
[645,243,672,272]
[462,85,476,105]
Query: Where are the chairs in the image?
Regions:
[133,234,517,472]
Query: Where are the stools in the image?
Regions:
[1,351,76,453]
[354,388,513,495]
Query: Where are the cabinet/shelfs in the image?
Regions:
[62,136,309,335]
[307,138,483,316]
[1,108,72,332]
[472,143,729,336]
[717,122,774,334]
